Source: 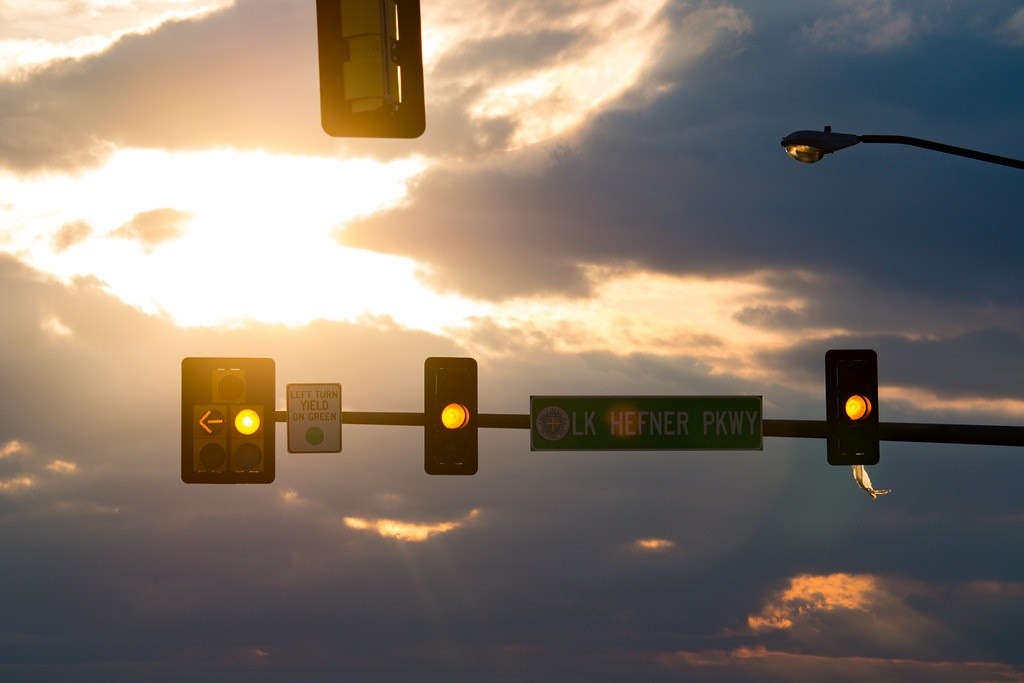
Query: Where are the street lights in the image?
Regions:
[780,123,1024,172]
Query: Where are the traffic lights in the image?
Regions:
[834,360,875,455]
[436,370,476,465]
[190,369,265,474]
